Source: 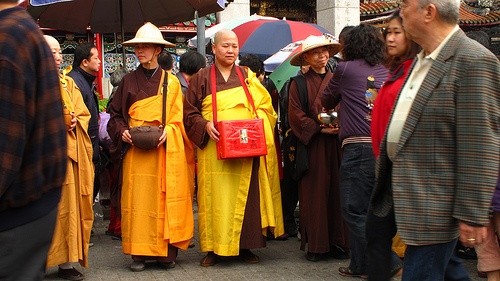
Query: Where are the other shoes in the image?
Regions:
[238,252,261,264]
[59,266,84,281]
[199,254,218,267]
[161,258,178,269]
[305,250,332,262]
[131,261,146,272]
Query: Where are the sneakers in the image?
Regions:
[339,264,367,279]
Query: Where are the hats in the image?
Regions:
[289,35,344,67]
[119,20,177,48]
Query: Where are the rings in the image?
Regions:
[466,238,476,241]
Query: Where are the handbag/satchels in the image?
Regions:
[217,118,267,161]
[276,131,308,180]
[126,126,165,149]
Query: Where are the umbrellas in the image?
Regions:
[266,45,305,95]
[16,0,232,70]
[188,14,279,47]
[263,41,302,71]
[228,16,335,56]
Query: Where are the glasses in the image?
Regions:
[306,49,330,57]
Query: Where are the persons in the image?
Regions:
[0,0,69,281]
[366,0,500,281]
[181,27,286,266]
[42,34,97,281]
[64,45,103,248]
[276,62,312,237]
[157,50,174,75]
[368,8,424,164]
[107,22,196,273]
[174,53,207,249]
[324,24,357,72]
[105,55,281,114]
[319,23,393,279]
[287,35,350,263]
